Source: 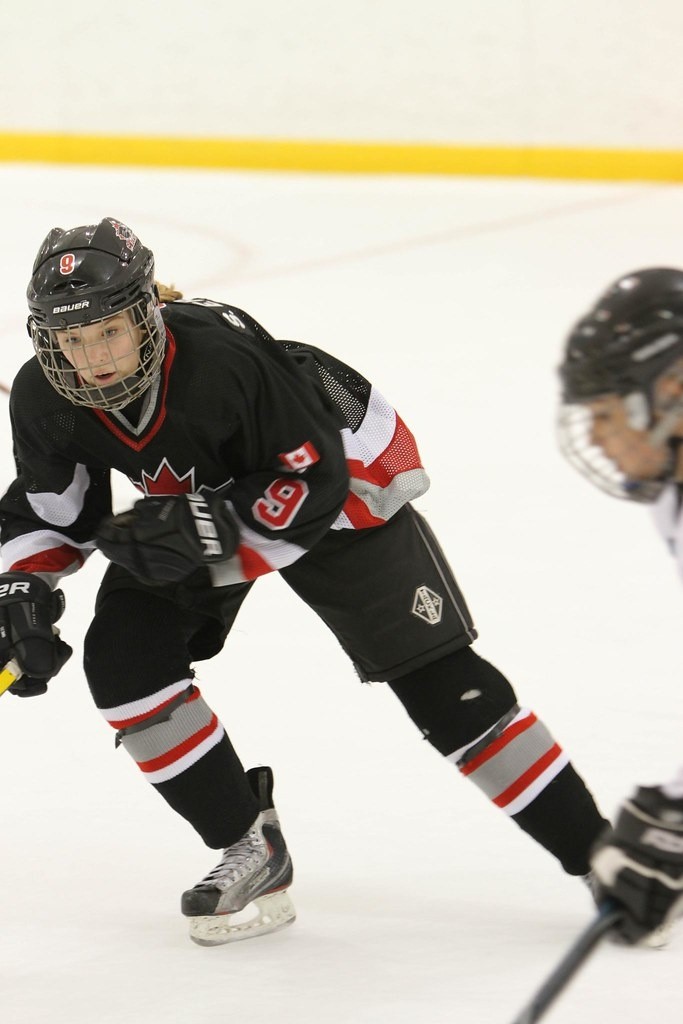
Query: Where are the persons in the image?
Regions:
[0,218,682,952]
[557,268,683,948]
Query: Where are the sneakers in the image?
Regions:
[181,766,296,947]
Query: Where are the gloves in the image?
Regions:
[0,571,72,697]
[95,493,239,586]
[589,786,683,945]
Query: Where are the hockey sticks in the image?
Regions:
[1,625,59,703]
[513,902,623,1024]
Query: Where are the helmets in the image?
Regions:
[556,268,683,504]
[27,217,166,411]
